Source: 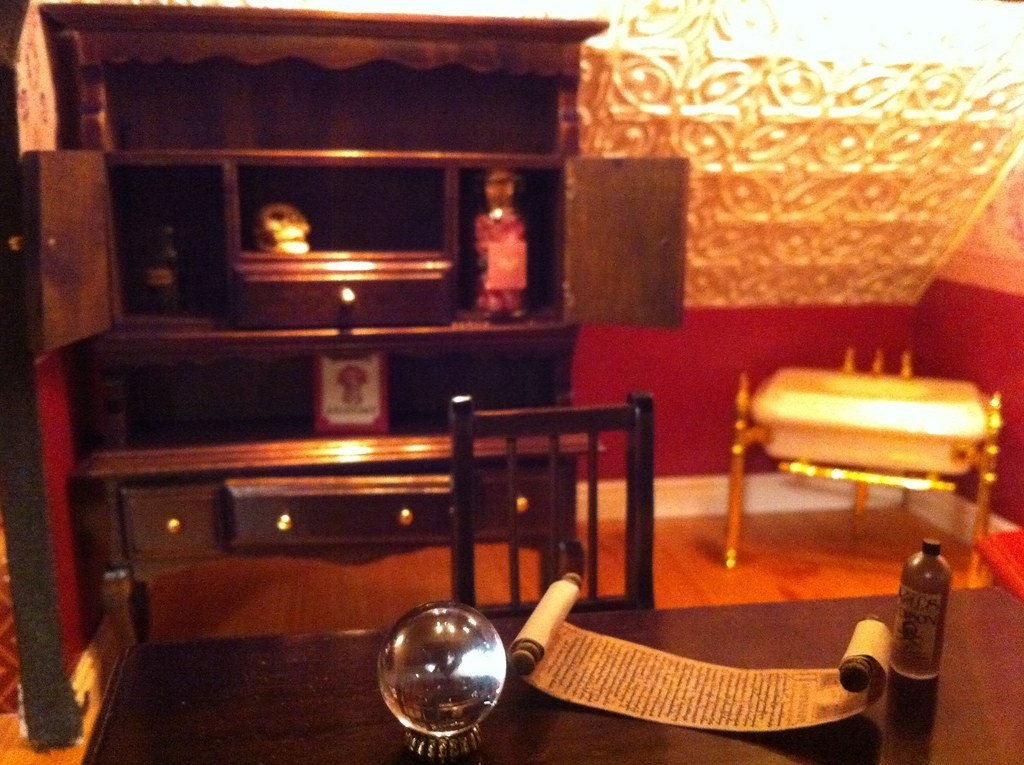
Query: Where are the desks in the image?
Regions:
[80,583,1024,765]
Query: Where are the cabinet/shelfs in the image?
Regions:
[18,2,694,647]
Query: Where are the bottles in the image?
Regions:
[474,168,529,322]
[888,538,951,678]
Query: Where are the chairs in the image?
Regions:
[448,391,655,618]
